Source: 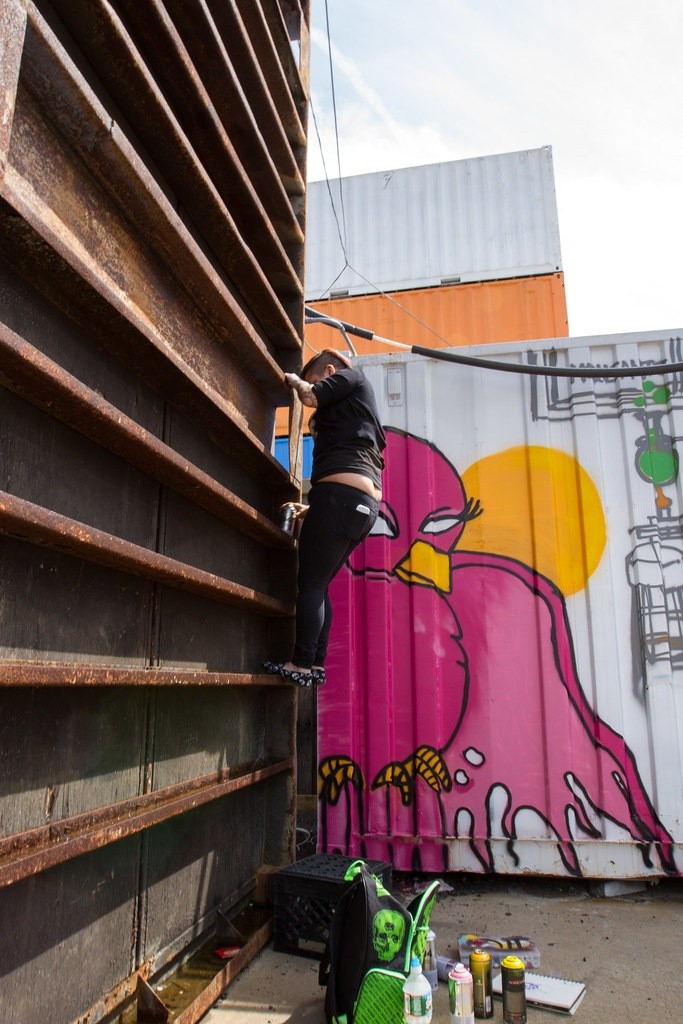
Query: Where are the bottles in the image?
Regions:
[438,955,470,983]
[279,503,297,537]
[469,948,493,1019]
[402,958,433,1024]
[422,929,438,990]
[447,963,474,1024]
[501,955,528,1024]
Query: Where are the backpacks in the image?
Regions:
[319,859,441,1024]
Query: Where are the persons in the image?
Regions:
[263,349,386,690]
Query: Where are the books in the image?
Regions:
[492,968,587,1014]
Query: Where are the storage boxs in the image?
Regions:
[277,141,683,878]
[276,852,393,959]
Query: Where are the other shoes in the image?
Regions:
[263,662,313,687]
[311,668,327,684]
